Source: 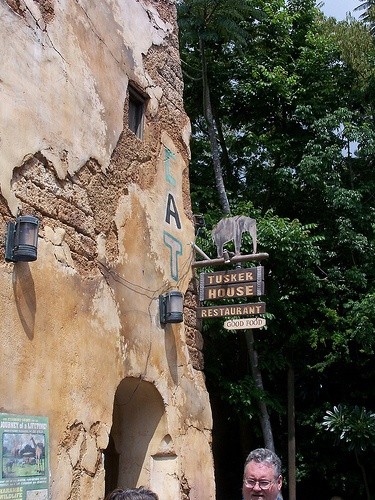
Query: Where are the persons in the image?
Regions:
[100,487,160,500]
[240,448,283,500]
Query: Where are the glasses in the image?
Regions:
[243,476,279,490]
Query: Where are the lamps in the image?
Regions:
[159,290,184,324]
[5,216,40,263]
